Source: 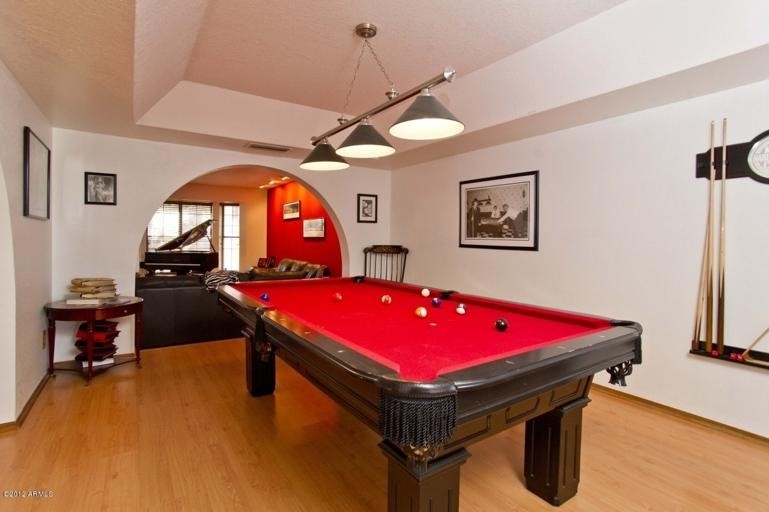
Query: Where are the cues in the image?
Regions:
[742,328,769,355]
[692,169,717,350]
[706,121,715,352]
[718,119,727,357]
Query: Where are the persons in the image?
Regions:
[87,177,115,203]
[495,203,519,237]
[491,205,501,219]
[467,197,481,237]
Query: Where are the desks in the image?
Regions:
[44,295,144,381]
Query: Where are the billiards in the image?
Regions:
[260,293,269,301]
[416,307,427,319]
[456,303,467,315]
[381,295,392,305]
[331,292,343,301]
[495,319,507,331]
[422,288,430,297]
[432,298,441,306]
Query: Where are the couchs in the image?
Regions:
[249,258,329,278]
[137,273,298,350]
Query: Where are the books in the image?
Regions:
[74,320,121,369]
[65,278,118,305]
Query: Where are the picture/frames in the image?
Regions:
[302,217,326,238]
[85,171,117,206]
[357,193,377,223]
[23,126,51,221]
[281,200,301,220]
[458,170,539,251]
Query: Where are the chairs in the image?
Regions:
[258,257,276,268]
[363,245,409,282]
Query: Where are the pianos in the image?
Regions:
[139,219,219,273]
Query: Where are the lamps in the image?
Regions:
[299,23,466,171]
